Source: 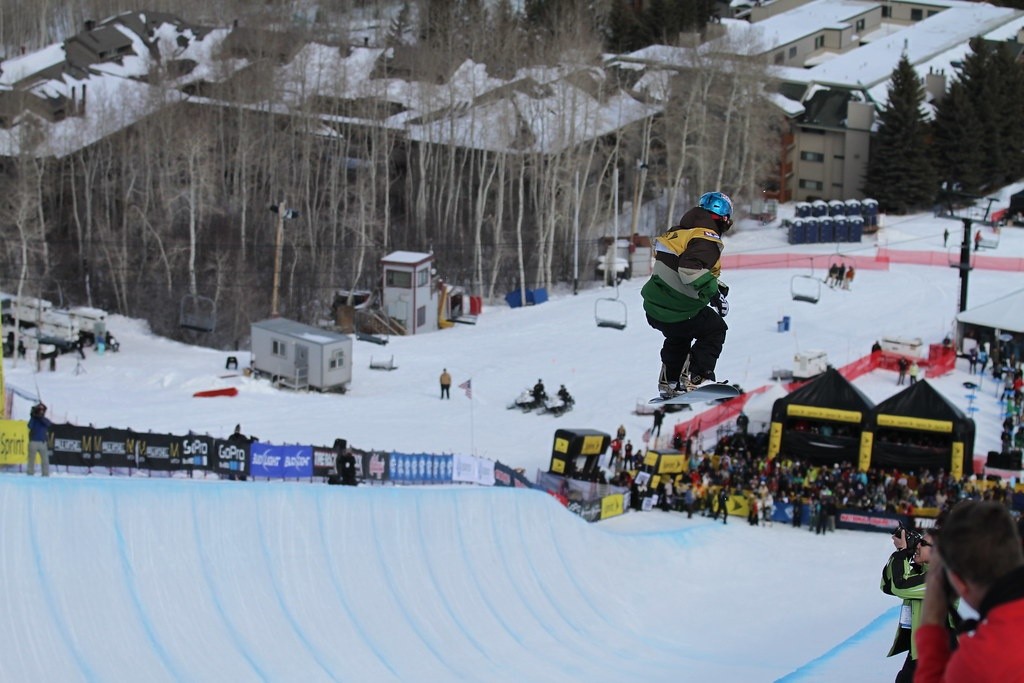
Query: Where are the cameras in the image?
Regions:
[893,526,922,558]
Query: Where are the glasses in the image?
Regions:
[920,539,932,547]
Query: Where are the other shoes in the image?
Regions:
[681,372,717,390]
[668,383,685,394]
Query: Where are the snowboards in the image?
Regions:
[648,383,740,405]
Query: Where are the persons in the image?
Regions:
[825,263,855,289]
[897,357,907,385]
[558,385,571,402]
[882,527,961,683]
[76,333,85,359]
[642,191,733,402]
[534,379,544,405]
[943,336,951,345]
[908,360,918,385]
[944,229,949,247]
[26,404,51,477]
[913,499,1024,683]
[975,230,981,251]
[967,330,1024,421]
[227,425,246,479]
[872,340,881,352]
[563,412,1024,535]
[651,408,666,437]
[440,368,452,399]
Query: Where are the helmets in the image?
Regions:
[699,191,733,222]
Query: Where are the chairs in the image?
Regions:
[226,357,237,370]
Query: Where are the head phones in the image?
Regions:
[720,216,732,232]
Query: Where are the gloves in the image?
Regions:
[710,292,728,317]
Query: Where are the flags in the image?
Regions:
[458,380,472,398]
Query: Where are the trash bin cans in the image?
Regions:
[784,316,790,330]
[778,321,785,332]
